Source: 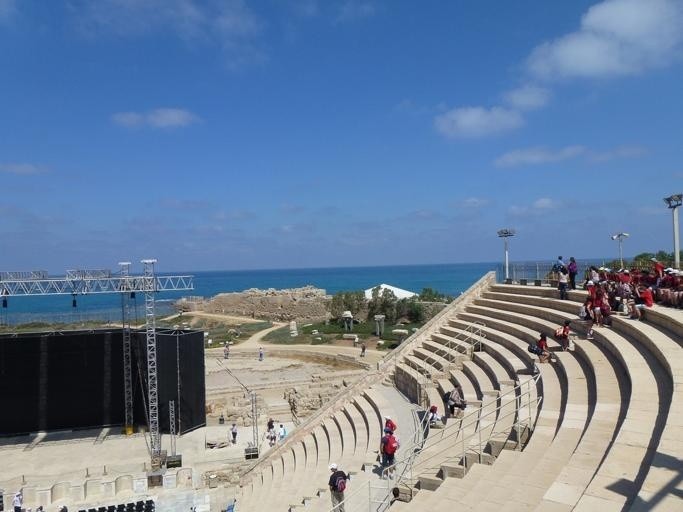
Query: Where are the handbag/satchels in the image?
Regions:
[527,344,538,353]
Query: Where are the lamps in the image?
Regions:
[1,287,141,309]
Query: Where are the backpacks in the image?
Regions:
[385,436,397,455]
[334,476,346,492]
[443,391,451,402]
[554,326,564,337]
[579,306,587,317]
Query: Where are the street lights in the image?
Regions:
[610,231,629,270]
[662,193,682,267]
[496,227,517,279]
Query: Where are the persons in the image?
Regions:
[227,344,230,357]
[360,342,366,357]
[448,385,468,417]
[226,498,236,512]
[391,487,404,506]
[380,427,396,480]
[189,507,196,512]
[218,412,288,448]
[224,345,229,359]
[384,415,397,432]
[258,346,264,361]
[527,256,682,364]
[427,405,441,422]
[328,463,347,512]
[1,487,69,512]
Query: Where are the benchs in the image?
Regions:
[204,276,682,511]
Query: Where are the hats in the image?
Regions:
[664,268,683,277]
[540,332,546,338]
[329,463,337,469]
[586,280,594,285]
[384,416,391,419]
[384,428,393,434]
[598,267,629,274]
